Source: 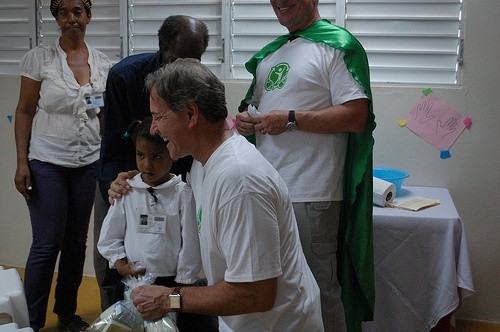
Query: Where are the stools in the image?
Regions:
[0,266,34,332]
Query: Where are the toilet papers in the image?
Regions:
[372,176,397,208]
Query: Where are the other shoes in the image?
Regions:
[56,315,88,332]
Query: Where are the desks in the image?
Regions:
[361,186,474,332]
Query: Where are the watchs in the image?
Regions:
[286,109,297,132]
[169,287,183,313]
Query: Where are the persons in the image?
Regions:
[97,118,205,332]
[235,0,377,332]
[93,15,209,313]
[130,57,325,332]
[14,0,113,332]
